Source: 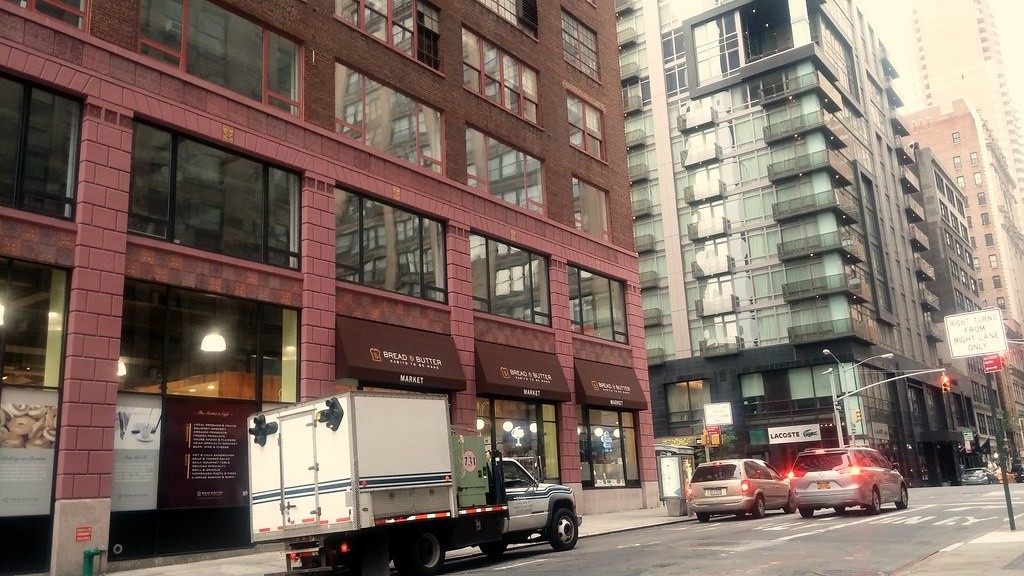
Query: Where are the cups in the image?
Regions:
[140,425,151,438]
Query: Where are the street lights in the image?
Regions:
[823,348,893,446]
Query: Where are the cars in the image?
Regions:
[961,467,1000,484]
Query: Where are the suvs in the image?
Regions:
[686,459,798,522]
[789,446,909,517]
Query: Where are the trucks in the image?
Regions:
[247,390,584,576]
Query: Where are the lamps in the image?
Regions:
[511,426,524,446]
[117,360,127,377]
[200,298,226,352]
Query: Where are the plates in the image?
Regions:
[136,434,155,442]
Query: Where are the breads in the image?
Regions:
[0,403,57,449]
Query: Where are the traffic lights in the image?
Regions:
[854,409,862,423]
[942,375,951,393]
[702,434,709,447]
